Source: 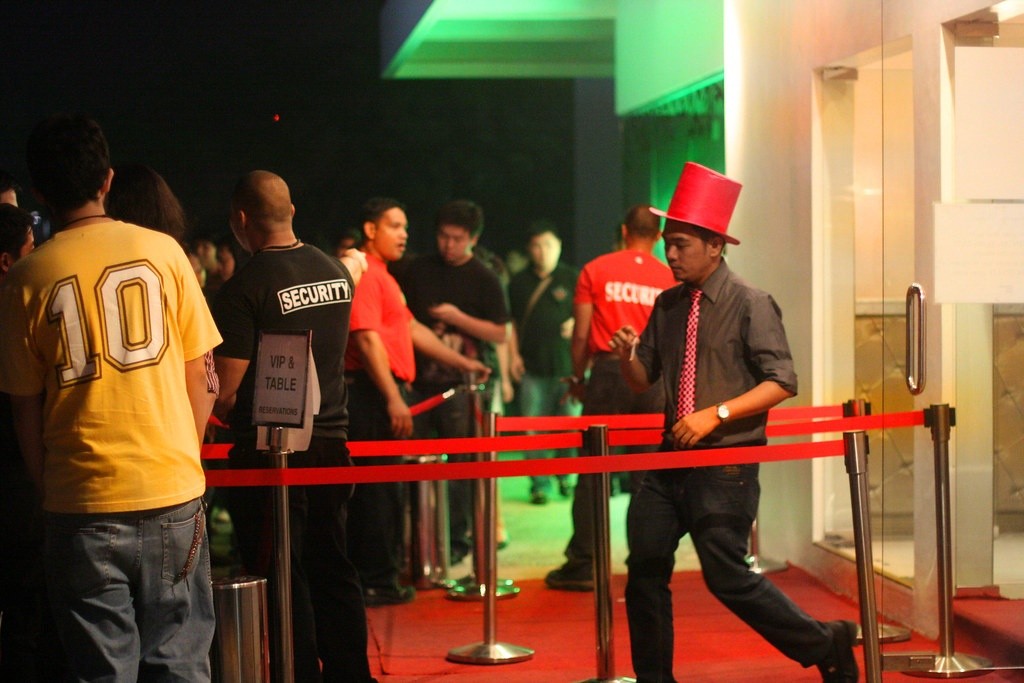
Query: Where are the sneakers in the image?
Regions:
[544,562,594,590]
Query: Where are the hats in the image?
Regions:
[647,160,742,248]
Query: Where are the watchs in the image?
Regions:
[716,402,731,425]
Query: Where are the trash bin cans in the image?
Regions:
[212,572,271,683]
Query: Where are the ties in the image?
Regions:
[676,290,705,423]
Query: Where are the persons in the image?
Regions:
[543,202,683,590]
[608,160,859,683]
[507,220,581,506]
[0,111,514,683]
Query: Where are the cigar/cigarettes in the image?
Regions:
[629,336,638,361]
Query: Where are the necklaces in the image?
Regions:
[252,238,301,256]
[49,213,108,231]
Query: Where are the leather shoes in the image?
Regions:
[815,620,858,683]
